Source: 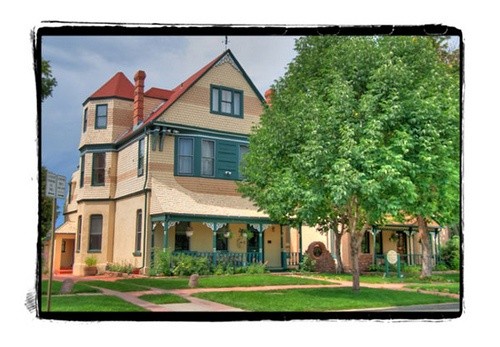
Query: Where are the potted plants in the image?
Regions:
[80,254,98,277]
